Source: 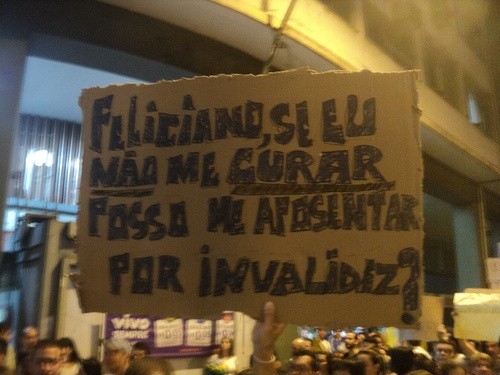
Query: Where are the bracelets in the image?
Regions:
[251,354,276,363]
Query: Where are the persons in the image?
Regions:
[203,301,500,375]
[0,321,172,375]
